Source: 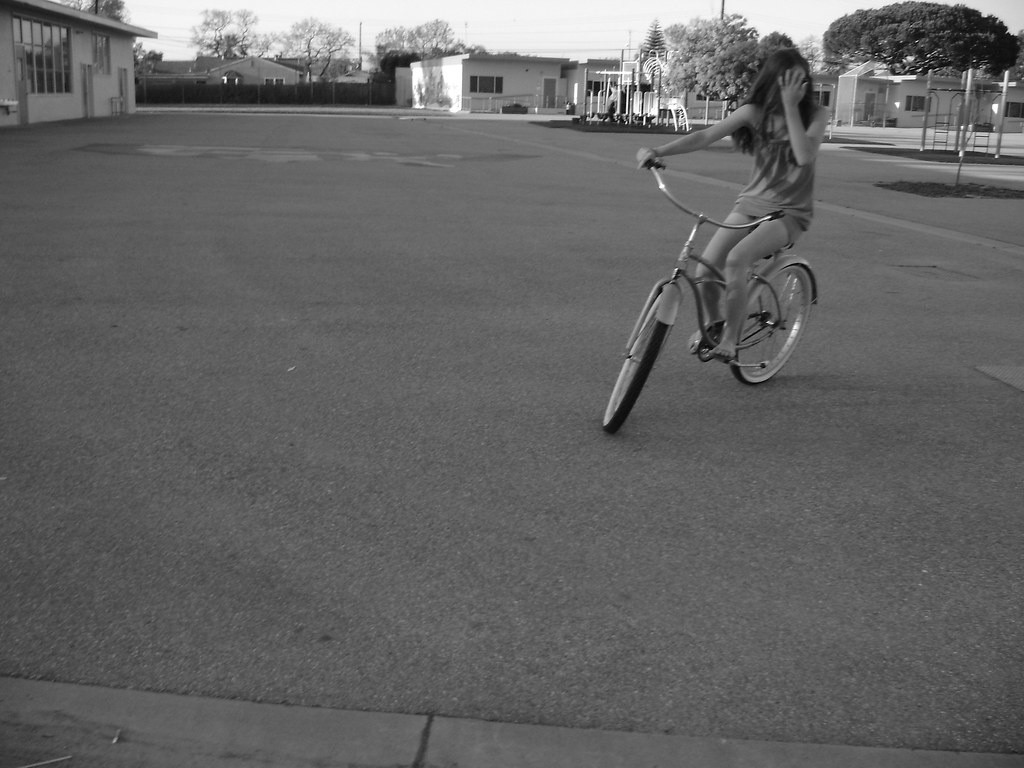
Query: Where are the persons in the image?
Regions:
[565,101,575,115]
[636,47,829,361]
[609,102,616,122]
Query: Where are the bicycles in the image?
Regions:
[601,158,819,435]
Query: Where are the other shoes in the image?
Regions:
[708,340,737,363]
[687,325,721,355]
